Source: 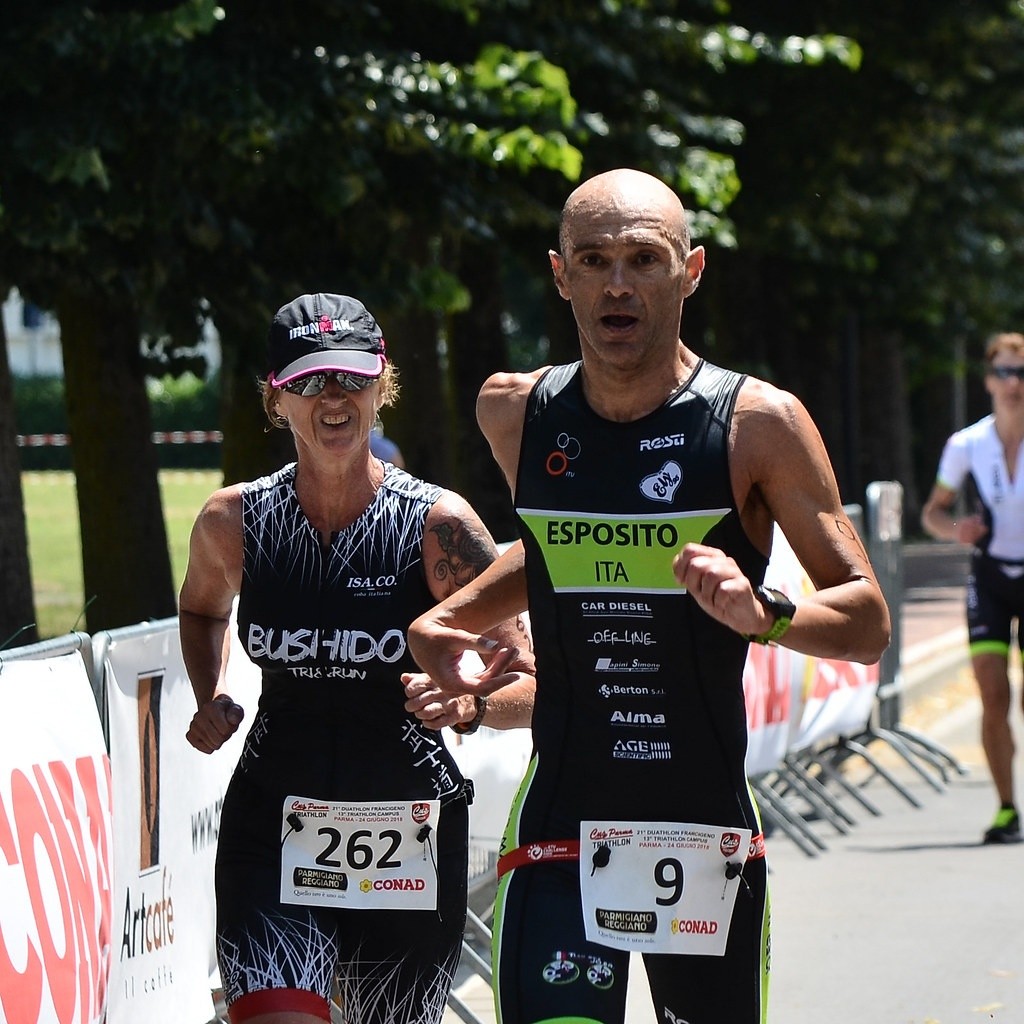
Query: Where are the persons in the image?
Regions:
[408,169,891,1024]
[178,293,536,1024]
[922,332,1024,841]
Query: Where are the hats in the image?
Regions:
[268,293,387,388]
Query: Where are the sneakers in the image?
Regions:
[985,802,1021,843]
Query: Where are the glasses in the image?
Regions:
[279,371,379,396]
[989,365,1024,380]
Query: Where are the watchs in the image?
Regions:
[451,697,488,737]
[742,585,796,648]
